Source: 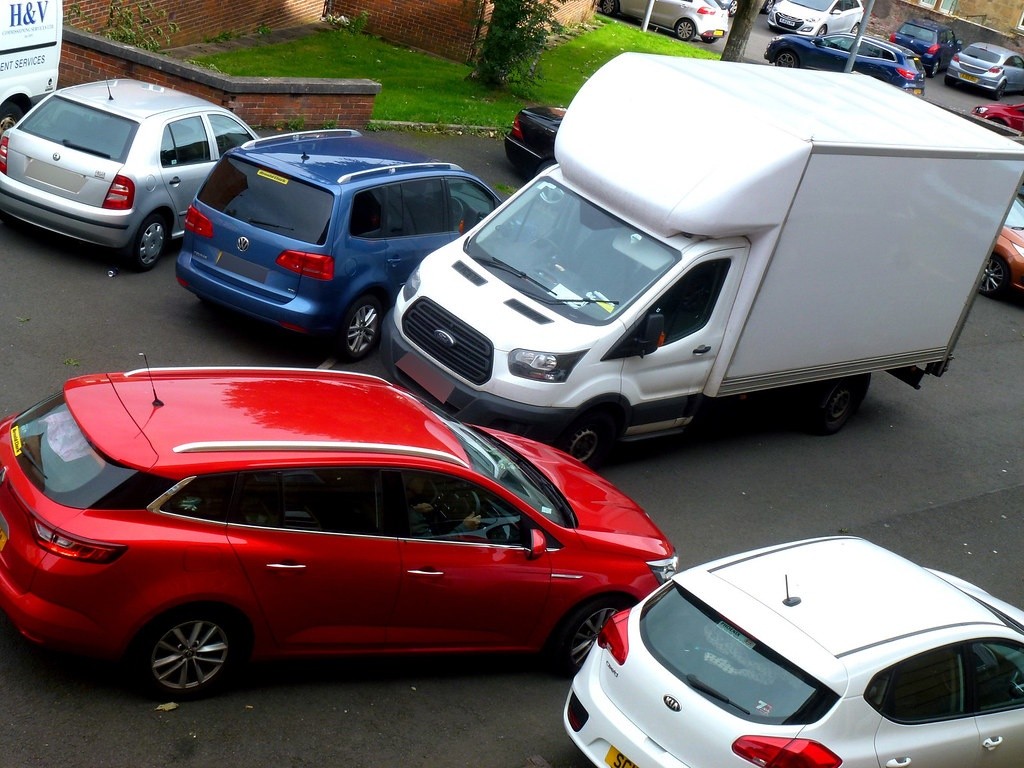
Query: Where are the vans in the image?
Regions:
[1,0,62,146]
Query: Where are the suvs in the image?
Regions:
[762,34,926,99]
[173,126,506,366]
[889,17,964,78]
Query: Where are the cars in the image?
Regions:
[766,0,868,39]
[504,107,570,183]
[978,195,1023,298]
[0,80,266,269]
[0,364,677,703]
[945,41,1023,101]
[969,104,1023,133]
[596,0,730,44]
[560,535,1024,768]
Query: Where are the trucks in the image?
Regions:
[379,53,1024,467]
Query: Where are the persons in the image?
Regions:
[385,471,482,540]
